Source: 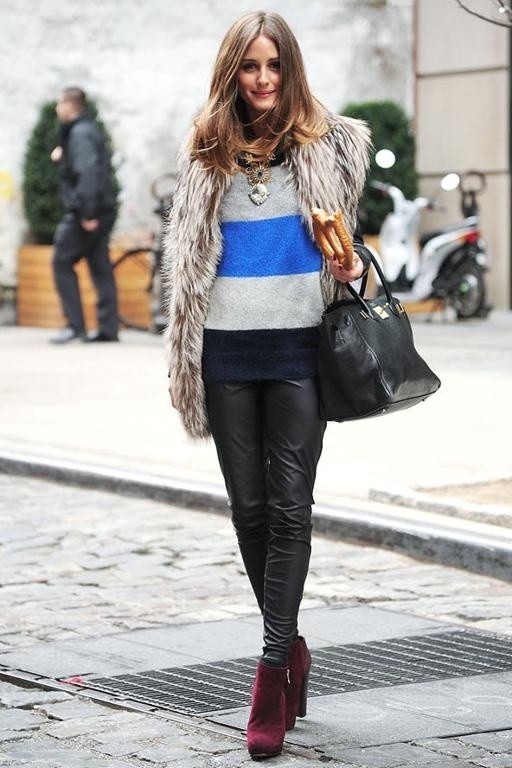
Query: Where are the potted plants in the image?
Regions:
[334,99,448,318]
[17,100,117,336]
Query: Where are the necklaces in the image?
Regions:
[242,150,276,207]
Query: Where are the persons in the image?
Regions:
[49,87,119,344]
[160,10,373,761]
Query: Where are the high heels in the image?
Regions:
[283,634,313,733]
[244,660,293,760]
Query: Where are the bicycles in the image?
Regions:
[107,173,178,335]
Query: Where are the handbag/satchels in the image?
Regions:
[313,240,443,424]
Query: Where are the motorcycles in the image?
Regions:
[364,148,491,322]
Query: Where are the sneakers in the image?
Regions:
[83,329,120,344]
[49,326,87,344]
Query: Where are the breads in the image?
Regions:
[311,209,353,269]
